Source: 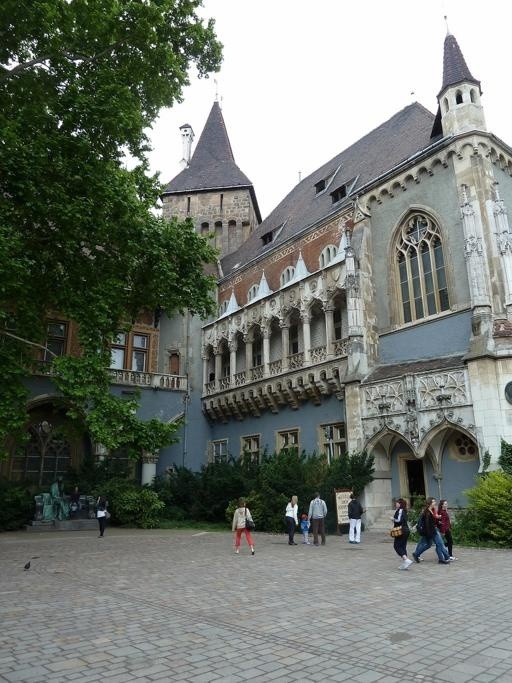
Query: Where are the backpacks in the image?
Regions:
[416,514,425,536]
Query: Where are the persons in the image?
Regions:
[71,484,81,511]
[436,498,458,560]
[96,494,107,535]
[415,496,448,564]
[51,475,71,521]
[348,493,363,542]
[412,498,459,562]
[389,497,412,569]
[300,513,311,544]
[285,495,299,545]
[308,491,328,545]
[232,502,256,554]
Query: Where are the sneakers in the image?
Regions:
[398,558,413,570]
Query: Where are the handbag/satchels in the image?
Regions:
[391,526,402,537]
[246,520,254,530]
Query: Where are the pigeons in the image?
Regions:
[23,560,31,571]
